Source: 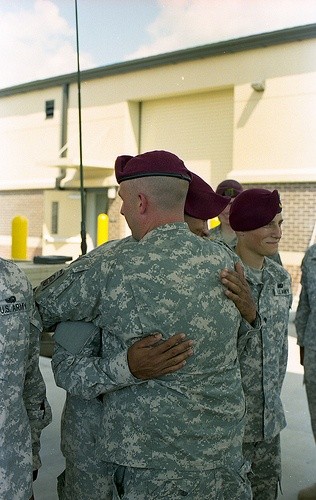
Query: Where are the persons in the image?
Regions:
[0,259,52,500]
[33,150,248,499]
[0,258,48,499]
[229,187,294,500]
[54,170,261,500]
[297,243,316,500]
[208,178,244,248]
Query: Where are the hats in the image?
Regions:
[216,179,243,197]
[114,150,192,183]
[228,188,282,232]
[184,170,231,220]
